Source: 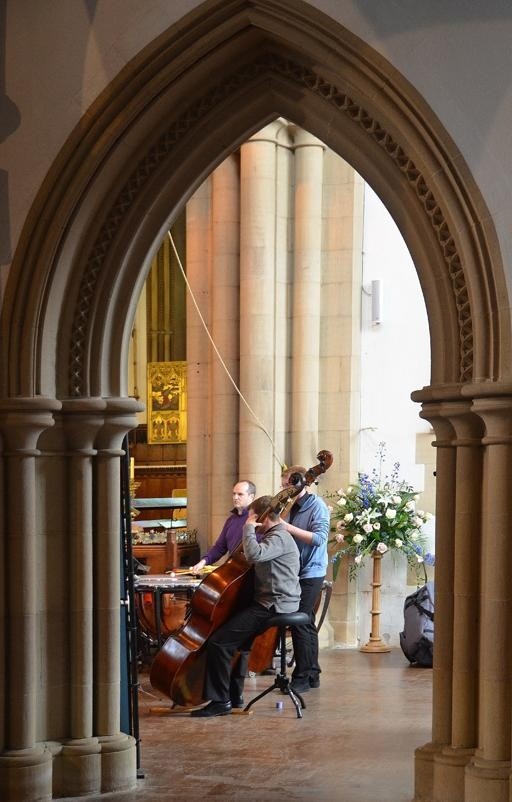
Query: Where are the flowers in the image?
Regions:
[329,457,430,572]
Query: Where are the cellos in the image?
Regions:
[148,451,334,707]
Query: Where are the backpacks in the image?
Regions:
[396,583,435,667]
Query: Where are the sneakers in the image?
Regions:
[281,679,311,696]
[309,677,321,689]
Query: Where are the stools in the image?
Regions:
[235,609,309,721]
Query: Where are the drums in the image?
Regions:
[129,572,202,643]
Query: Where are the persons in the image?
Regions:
[277,464,331,696]
[187,479,260,572]
[188,494,301,718]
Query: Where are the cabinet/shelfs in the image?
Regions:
[123,531,198,644]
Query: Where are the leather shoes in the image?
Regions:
[190,699,233,719]
[230,694,245,709]
